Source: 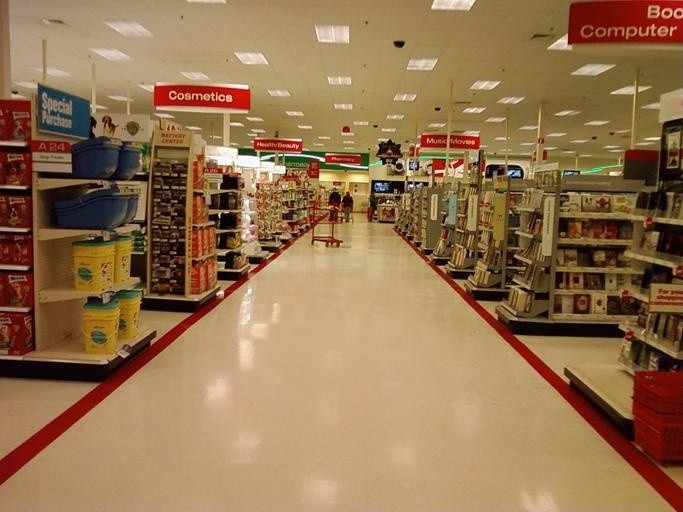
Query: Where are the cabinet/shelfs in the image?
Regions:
[210,143,320,280]
[93,114,223,314]
[561,168,680,438]
[2,93,160,381]
[486,168,644,338]
[462,170,535,302]
[393,167,485,279]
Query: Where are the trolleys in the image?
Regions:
[310,200,354,248]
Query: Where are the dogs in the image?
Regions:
[101,115,120,138]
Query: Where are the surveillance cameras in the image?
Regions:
[434,107,441,111]
[368,148,370,150]
[374,125,377,128]
[392,40,404,49]
[405,140,408,142]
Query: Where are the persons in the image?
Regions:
[319,186,325,198]
[342,192,353,222]
[367,189,376,218]
[369,191,379,221]
[328,188,341,223]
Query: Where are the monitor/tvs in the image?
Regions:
[409,162,418,170]
[564,170,580,176]
[508,169,522,177]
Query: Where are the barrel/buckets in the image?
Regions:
[71,235,144,356]
